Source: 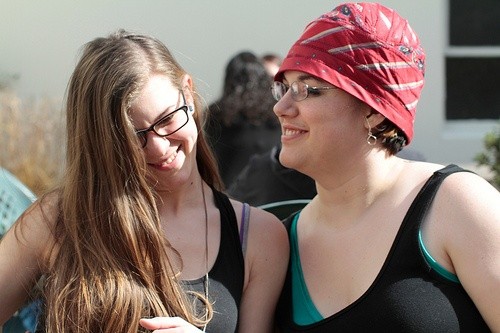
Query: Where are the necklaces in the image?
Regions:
[203,177,210,333]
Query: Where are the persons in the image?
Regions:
[0,30,290,333]
[262,54,282,75]
[203,51,317,221]
[272,3,500,331]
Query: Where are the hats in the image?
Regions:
[274,2,426,146]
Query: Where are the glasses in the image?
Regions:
[270,80,337,102]
[134,85,189,151]
